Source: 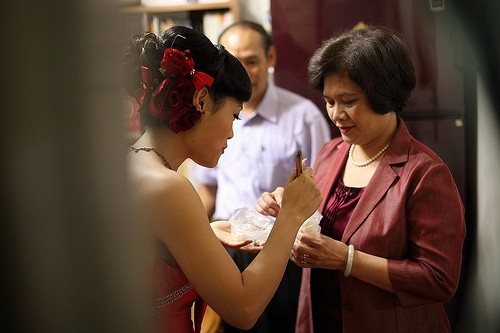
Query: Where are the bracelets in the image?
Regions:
[344,244,354,277]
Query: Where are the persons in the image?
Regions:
[122,20,331,333]
[257,25,466,333]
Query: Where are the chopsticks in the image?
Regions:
[295,149,302,177]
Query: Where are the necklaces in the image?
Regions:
[349,145,390,167]
[126,145,173,169]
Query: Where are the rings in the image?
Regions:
[300,254,309,264]
[311,175,314,180]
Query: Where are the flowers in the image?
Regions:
[149,48,204,134]
